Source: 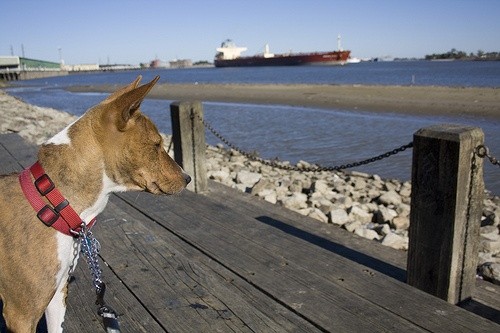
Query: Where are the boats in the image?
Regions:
[215,51,347,68]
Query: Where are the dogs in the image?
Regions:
[1,73,192,333]
